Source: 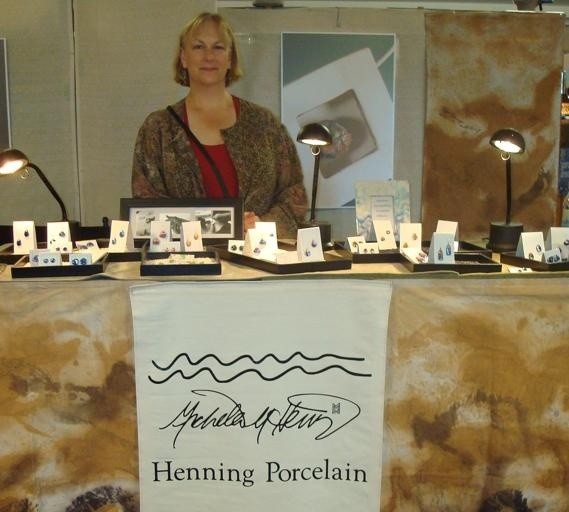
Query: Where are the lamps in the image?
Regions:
[295,123,335,250]
[486,127,526,253]
[0,150,80,249]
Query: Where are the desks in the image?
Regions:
[0,238,569,510]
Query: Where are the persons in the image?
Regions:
[126,6,310,238]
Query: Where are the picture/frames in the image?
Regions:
[119,197,244,248]
[279,31,397,211]
